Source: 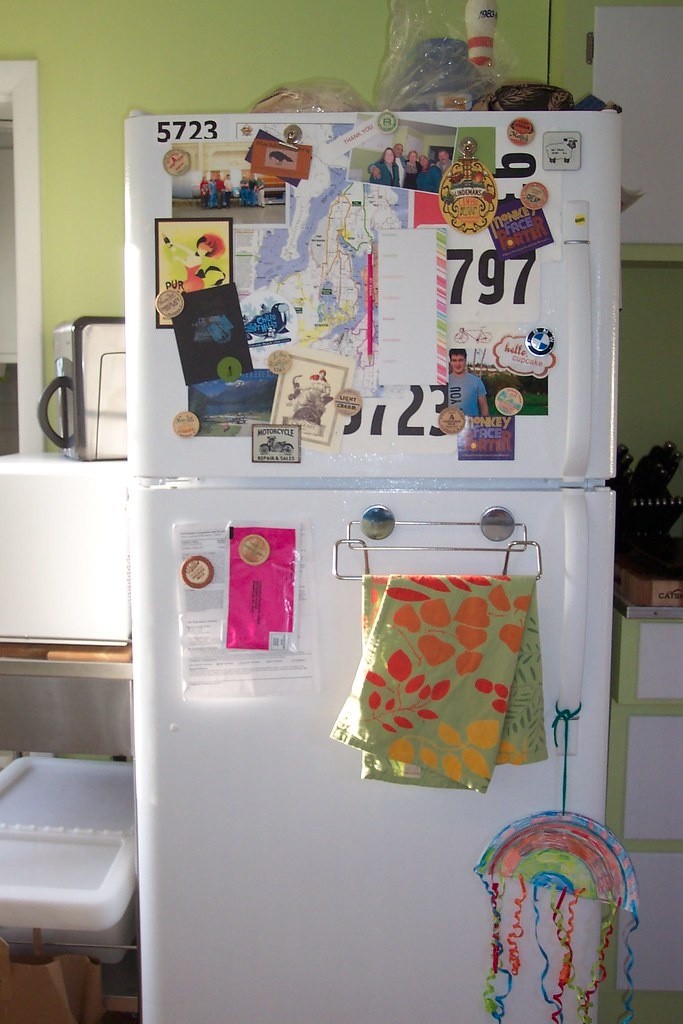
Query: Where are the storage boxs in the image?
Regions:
[1,451,136,647]
[1,759,139,964]
[599,569,683,1024]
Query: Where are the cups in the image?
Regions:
[399,37,484,111]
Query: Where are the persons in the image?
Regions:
[448,348,490,416]
[367,143,452,193]
[199,174,267,208]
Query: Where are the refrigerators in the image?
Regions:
[119,109,624,1024]
